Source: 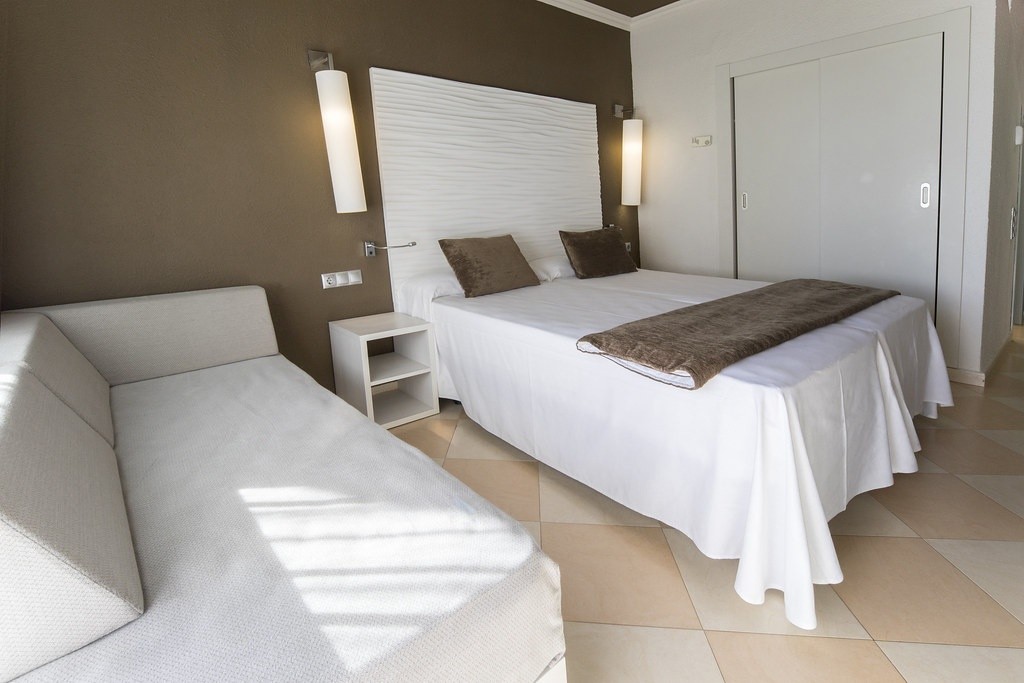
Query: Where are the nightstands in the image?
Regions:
[329,312,440,430]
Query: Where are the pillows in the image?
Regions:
[398,263,551,302]
[437,234,541,298]
[558,227,638,280]
[529,254,576,281]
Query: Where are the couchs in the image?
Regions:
[0,284,566,683]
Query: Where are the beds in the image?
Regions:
[368,65,954,631]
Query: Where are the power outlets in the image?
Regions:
[625,242,631,251]
[321,270,363,290]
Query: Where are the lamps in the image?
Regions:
[613,103,643,206]
[307,48,368,214]
